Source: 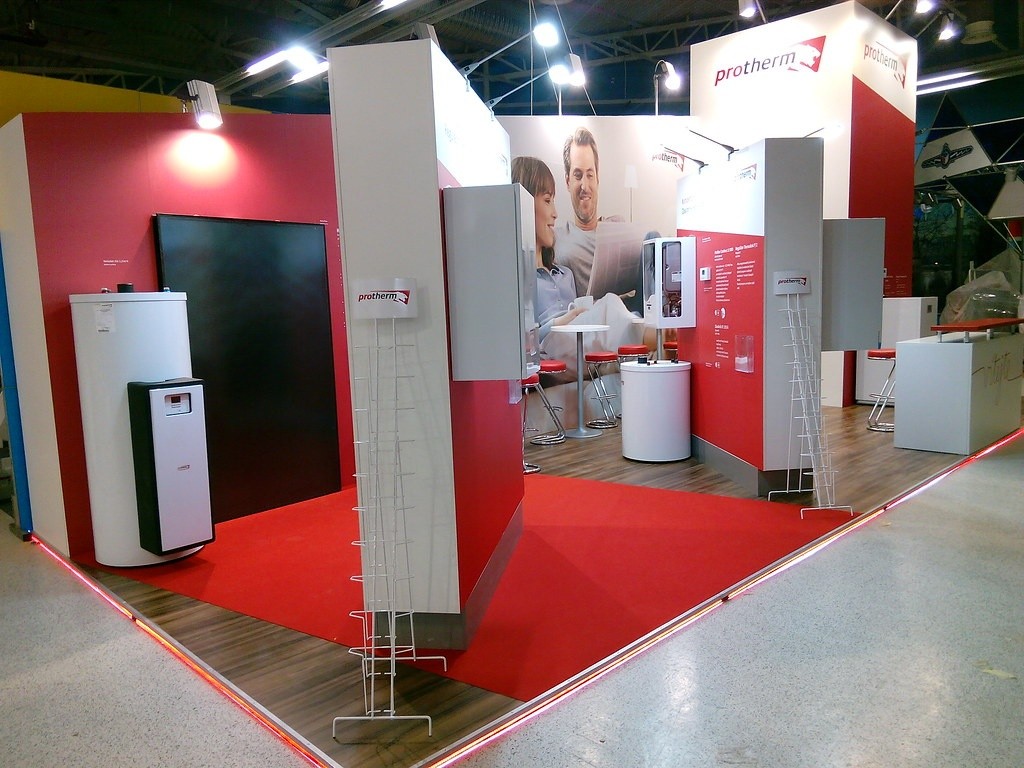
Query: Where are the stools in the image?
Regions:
[520,360,567,474]
[584,341,678,429]
[866,348,896,433]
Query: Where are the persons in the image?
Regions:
[511,127,676,382]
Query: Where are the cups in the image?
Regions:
[638,356,647,363]
[117,283,133,292]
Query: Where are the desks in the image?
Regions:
[550,325,610,439]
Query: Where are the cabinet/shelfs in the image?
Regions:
[443,182,541,381]
[893,317,1024,456]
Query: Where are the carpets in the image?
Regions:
[76,475,859,696]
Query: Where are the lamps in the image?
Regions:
[739,0,767,25]
[177,80,223,129]
[914,10,958,41]
[460,17,588,112]
[623,165,639,223]
[878,0,935,20]
[654,58,681,117]
